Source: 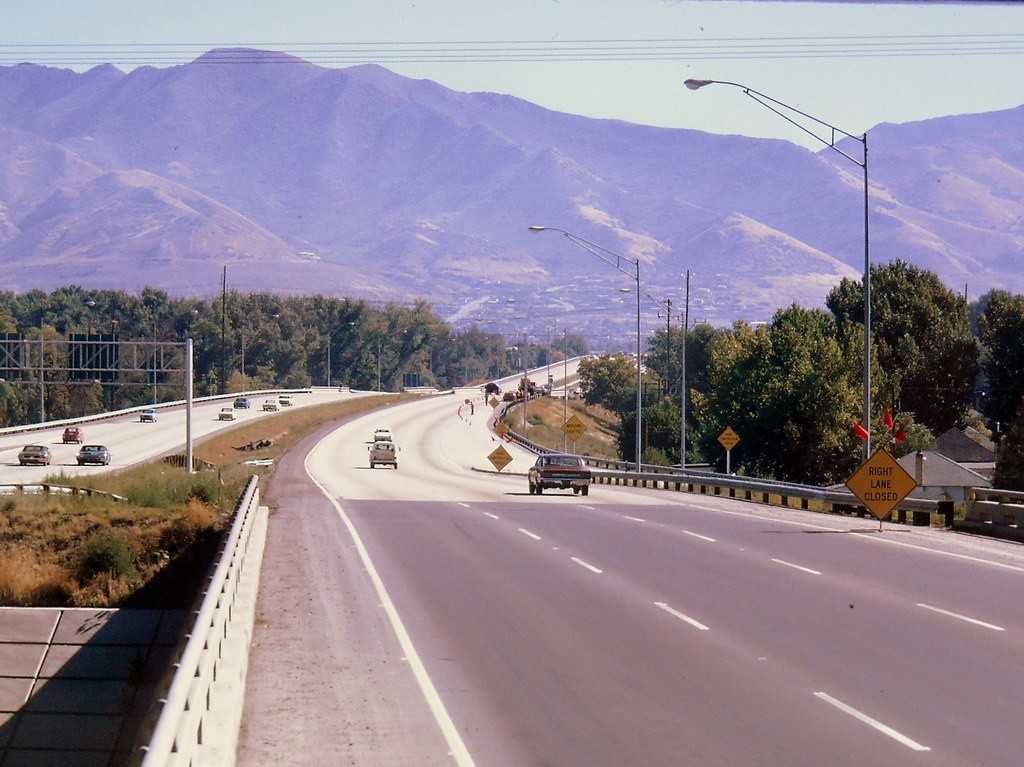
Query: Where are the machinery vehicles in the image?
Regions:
[518,378,532,392]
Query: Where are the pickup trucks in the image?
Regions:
[368,441,401,469]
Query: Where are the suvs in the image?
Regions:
[140,408,158,422]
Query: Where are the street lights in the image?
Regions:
[464,336,489,385]
[377,329,408,392]
[617,287,672,413]
[684,77,871,464]
[528,225,640,471]
[39,300,95,422]
[152,308,198,404]
[326,321,354,386]
[428,336,456,386]
[507,298,568,453]
[240,314,281,391]
[528,305,552,397]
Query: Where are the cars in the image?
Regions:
[219,407,235,421]
[18,444,52,466]
[484,383,502,395]
[263,400,279,411]
[374,429,393,442]
[62,427,84,444]
[528,453,591,495]
[76,444,111,465]
[278,395,293,407]
[234,397,251,409]
[503,391,517,401]
